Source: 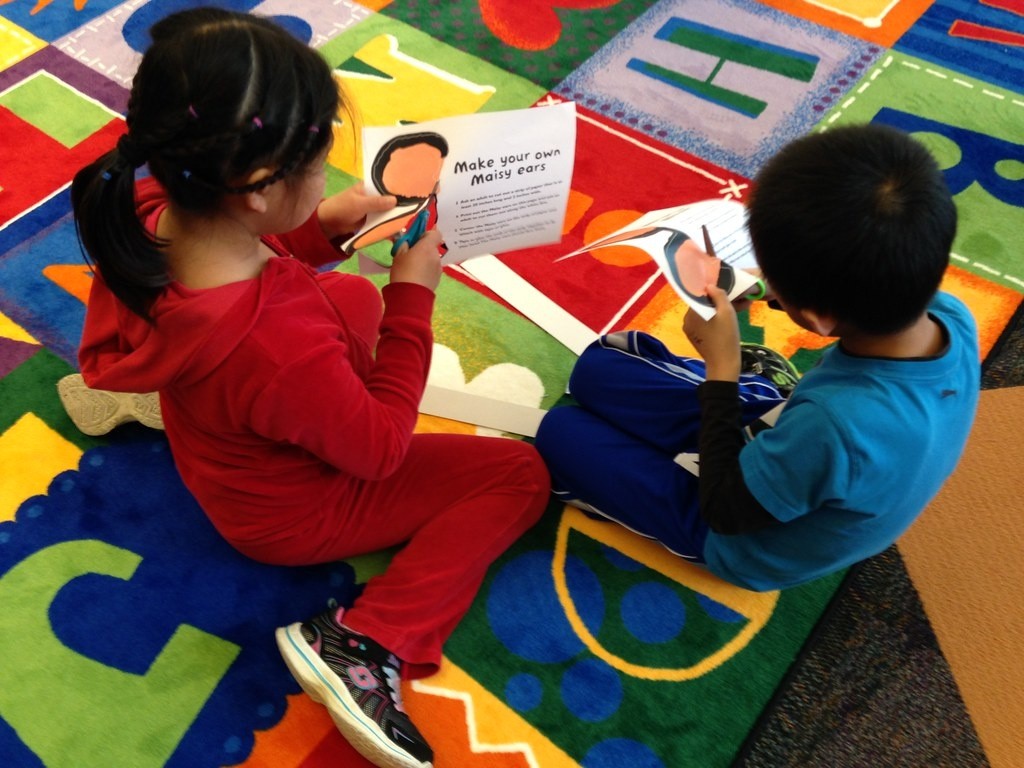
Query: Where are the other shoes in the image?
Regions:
[737,341,803,397]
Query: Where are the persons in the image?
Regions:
[67,6,551,768]
[536,119,980,594]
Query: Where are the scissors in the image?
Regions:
[702,224,765,300]
[391,181,439,257]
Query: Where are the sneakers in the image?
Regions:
[55,365,181,439]
[276,606,440,767]
